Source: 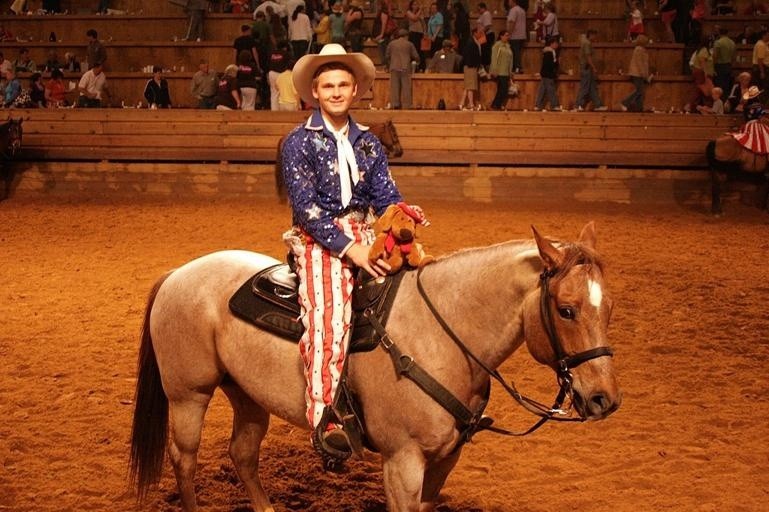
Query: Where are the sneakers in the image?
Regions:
[317,423,353,459]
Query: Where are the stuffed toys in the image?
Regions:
[369,201,436,272]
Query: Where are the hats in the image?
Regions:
[291,42,377,110]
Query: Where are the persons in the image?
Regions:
[740,85,765,135]
[0,0,769,113]
[281,62,405,469]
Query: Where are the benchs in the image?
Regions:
[0,0,764,163]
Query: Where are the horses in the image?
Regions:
[129,218,623,512]
[274,115,405,206]
[0,116,23,198]
